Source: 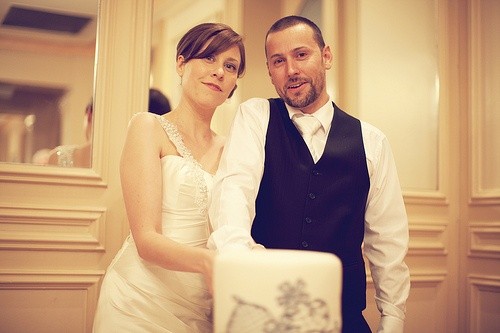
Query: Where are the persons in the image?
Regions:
[207,15,411,332]
[148,88,172,115]
[44,101,93,168]
[92,23,246,333]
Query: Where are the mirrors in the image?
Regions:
[0,0,99,169]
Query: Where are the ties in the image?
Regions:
[294,116,321,150]
